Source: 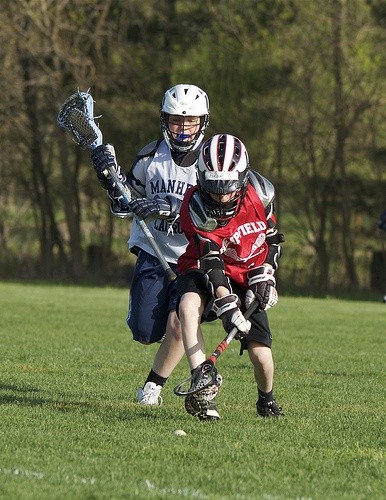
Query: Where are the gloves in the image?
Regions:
[245,265,279,313]
[90,143,124,191]
[211,293,252,340]
[129,195,182,221]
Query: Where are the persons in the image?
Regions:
[91,85,222,407]
[169,134,287,416]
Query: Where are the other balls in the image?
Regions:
[174,430,187,436]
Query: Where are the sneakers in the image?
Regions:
[256,397,285,417]
[188,373,222,400]
[136,381,163,406]
[183,396,217,421]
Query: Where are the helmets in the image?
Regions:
[194,134,250,227]
[160,83,210,152]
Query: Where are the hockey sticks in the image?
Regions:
[57,91,177,280]
[173,298,260,404]
